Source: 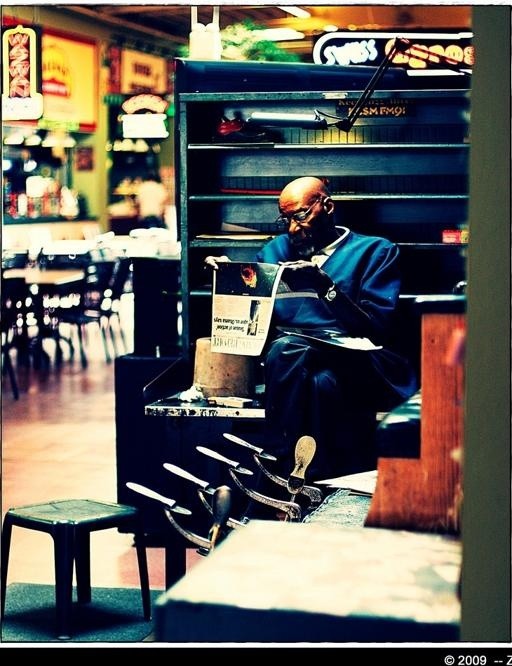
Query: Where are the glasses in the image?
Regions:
[275,198,321,232]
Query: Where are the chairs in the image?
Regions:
[1,247,133,400]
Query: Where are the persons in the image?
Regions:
[198,177,402,556]
[21,148,40,176]
[6,157,34,194]
[132,169,168,225]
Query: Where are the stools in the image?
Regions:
[2,499,153,641]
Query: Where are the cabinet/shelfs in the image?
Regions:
[176,86,471,361]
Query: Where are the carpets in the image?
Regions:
[4,580,165,642]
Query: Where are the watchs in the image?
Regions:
[321,282,340,303]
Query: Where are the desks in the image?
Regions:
[141,385,265,591]
